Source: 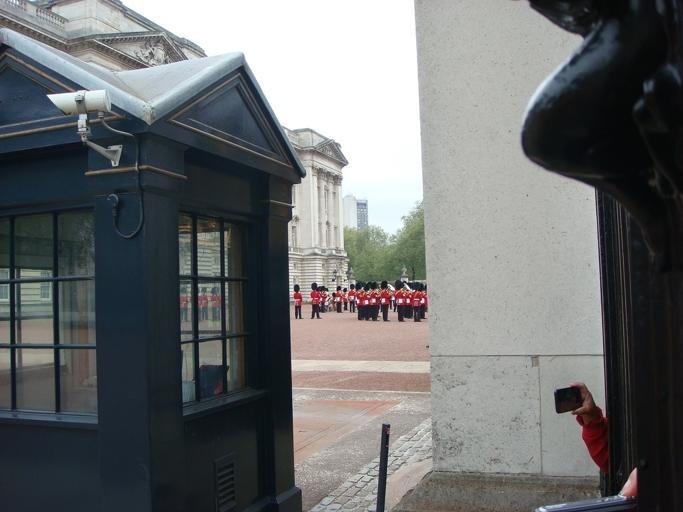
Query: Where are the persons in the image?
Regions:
[181,286,220,321]
[568,381,609,473]
[293,284,303,319]
[311,280,427,322]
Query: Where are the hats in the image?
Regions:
[311,280,427,297]
[180,287,219,294]
[294,285,300,292]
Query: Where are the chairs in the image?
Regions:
[199,364,229,396]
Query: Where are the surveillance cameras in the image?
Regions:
[46,88,112,116]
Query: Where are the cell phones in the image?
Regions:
[553,386,583,414]
